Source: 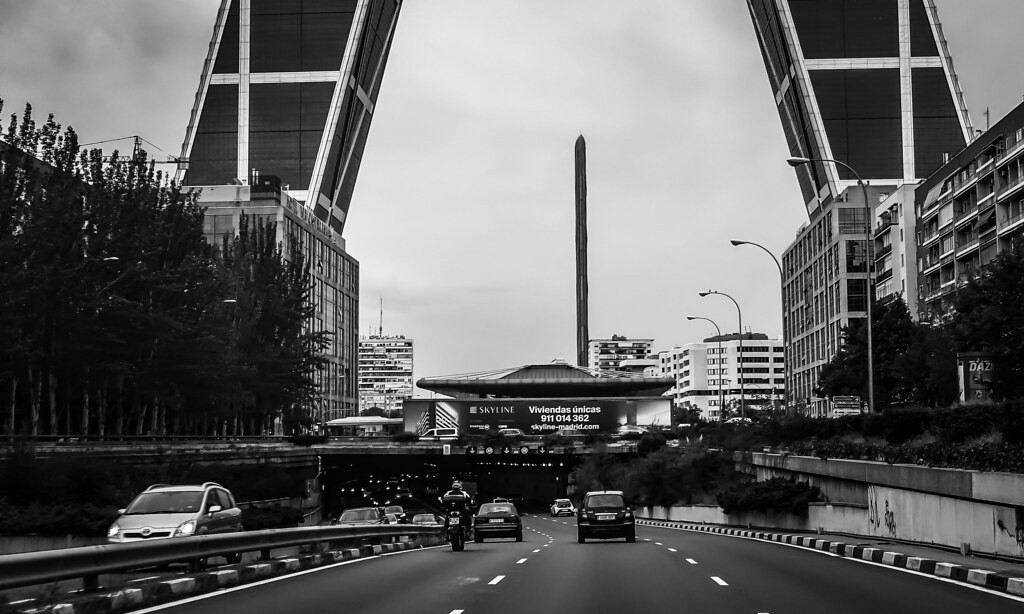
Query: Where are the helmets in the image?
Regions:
[452,481,463,489]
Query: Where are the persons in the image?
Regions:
[441,480,473,541]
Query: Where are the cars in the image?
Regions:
[574,490,636,543]
[360,473,441,527]
[473,502,523,541]
[724,418,752,423]
[549,498,574,517]
[497,428,525,435]
[330,506,391,546]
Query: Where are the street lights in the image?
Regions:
[786,157,874,414]
[699,292,747,424]
[729,238,791,410]
[687,316,723,424]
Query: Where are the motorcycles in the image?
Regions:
[443,495,471,551]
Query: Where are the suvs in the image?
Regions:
[107,481,245,571]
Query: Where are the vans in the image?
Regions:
[418,428,460,441]
[357,432,390,441]
